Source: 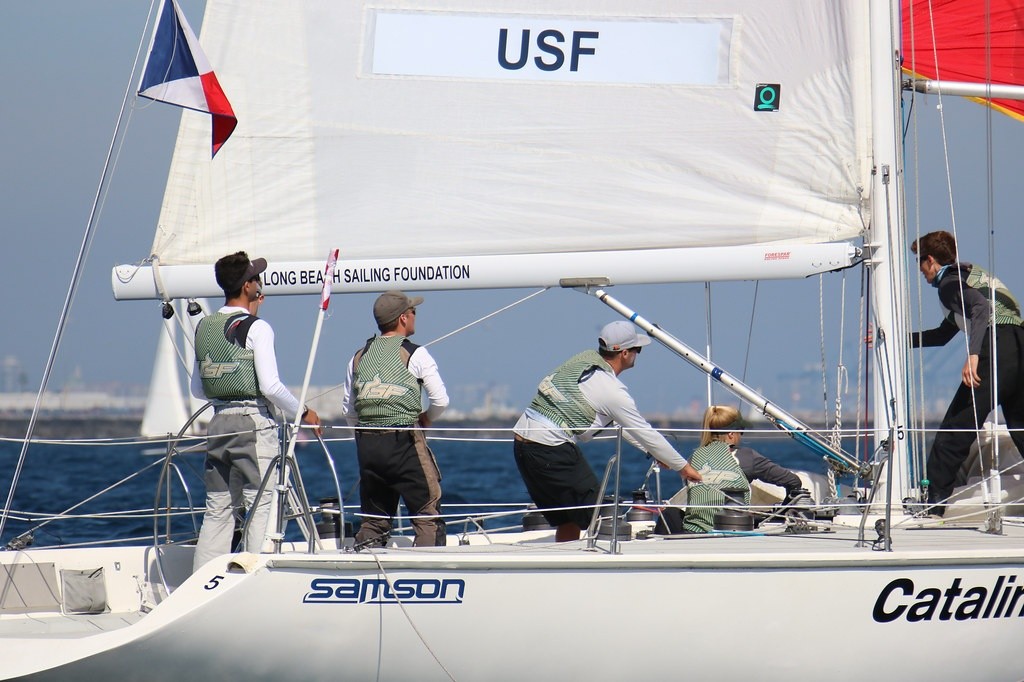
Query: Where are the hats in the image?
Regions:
[373,292,424,325]
[712,410,754,435]
[598,321,651,351]
[215,251,267,291]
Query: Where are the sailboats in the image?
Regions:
[2,0,1023,680]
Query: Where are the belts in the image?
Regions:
[514,434,532,443]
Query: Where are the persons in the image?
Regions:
[909,231,1024,519]
[342,291,450,547]
[191,251,323,575]
[513,321,703,543]
[654,406,802,536]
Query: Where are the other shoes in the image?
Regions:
[902,498,945,516]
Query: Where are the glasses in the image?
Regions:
[617,346,642,355]
[249,275,259,281]
[916,254,930,266]
[405,308,415,316]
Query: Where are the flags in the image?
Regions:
[137,0,239,160]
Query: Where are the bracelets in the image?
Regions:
[302,405,308,419]
